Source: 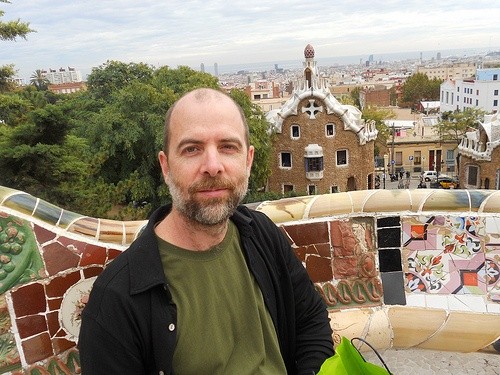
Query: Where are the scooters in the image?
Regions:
[389,172,398,182]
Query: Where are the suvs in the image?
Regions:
[430,177,460,189]
[419,171,452,182]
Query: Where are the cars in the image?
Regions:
[435,179,459,189]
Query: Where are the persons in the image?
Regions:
[447,175,463,190]
[397,167,429,189]
[75,85,337,375]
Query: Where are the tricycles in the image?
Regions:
[417,182,427,188]
